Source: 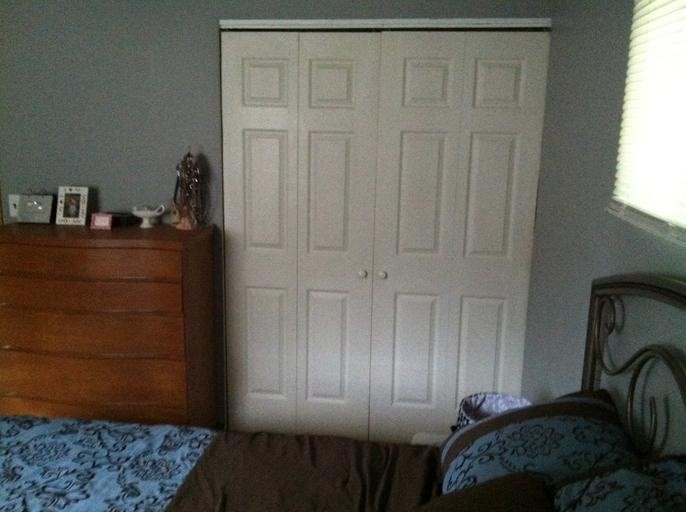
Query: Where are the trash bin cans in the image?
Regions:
[456,392,532,432]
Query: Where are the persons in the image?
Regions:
[64,198,77,217]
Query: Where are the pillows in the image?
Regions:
[436,387,635,480]
[412,469,552,508]
[550,457,684,509]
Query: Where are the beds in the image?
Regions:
[0,259,683,512]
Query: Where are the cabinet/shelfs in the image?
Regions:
[0,223,213,429]
[217,19,553,445]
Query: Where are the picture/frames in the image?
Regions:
[56,185,88,226]
